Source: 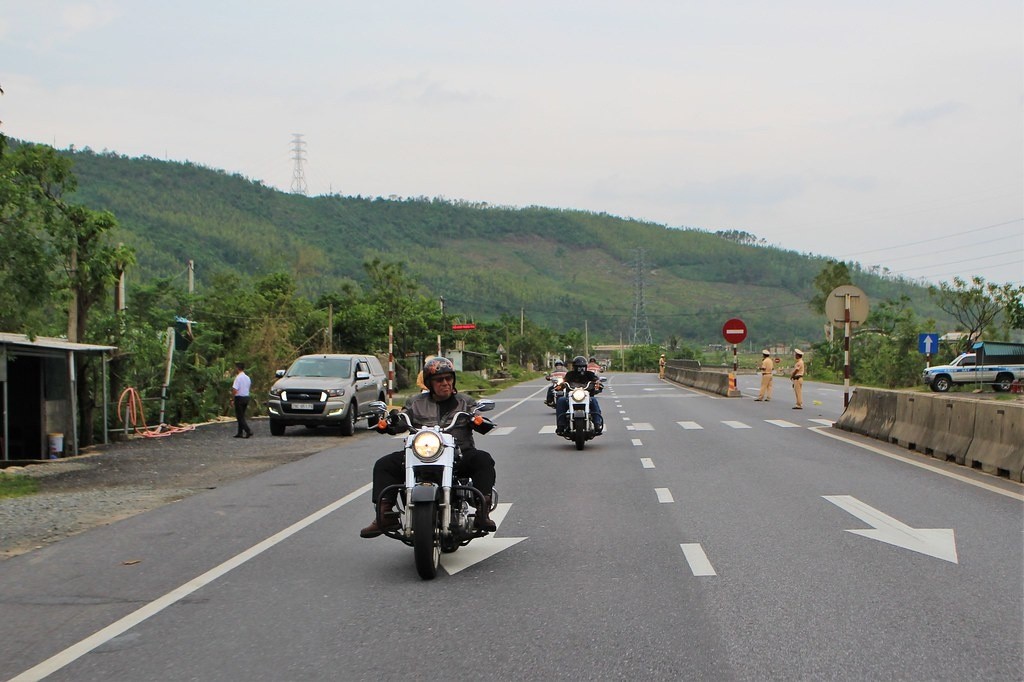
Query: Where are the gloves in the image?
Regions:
[556,386,561,390]
[595,381,600,389]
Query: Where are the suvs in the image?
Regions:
[268,354,388,437]
[922,353,1024,392]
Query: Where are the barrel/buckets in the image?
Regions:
[384,406,402,415]
[49,433,64,452]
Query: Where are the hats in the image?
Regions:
[763,350,770,355]
[661,354,664,357]
[795,349,804,355]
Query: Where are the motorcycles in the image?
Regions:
[587,363,606,376]
[551,377,607,451]
[543,372,566,409]
[367,400,498,581]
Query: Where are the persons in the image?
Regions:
[543,360,569,404]
[359,357,496,539]
[555,355,602,434]
[587,357,602,389]
[232,363,254,439]
[658,354,666,379]
[753,350,774,401]
[416,355,435,393]
[790,348,804,409]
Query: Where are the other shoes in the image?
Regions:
[595,423,600,431]
[754,398,762,401]
[556,426,564,433]
[243,431,253,438]
[765,399,769,401]
[792,407,803,409]
[233,434,243,438]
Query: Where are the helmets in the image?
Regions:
[572,356,588,373]
[423,357,456,390]
[554,360,564,367]
[590,357,595,362]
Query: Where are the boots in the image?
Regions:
[360,501,398,538]
[473,505,496,531]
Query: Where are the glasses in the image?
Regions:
[556,363,562,366]
[578,366,586,372]
[430,376,452,382]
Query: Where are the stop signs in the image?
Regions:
[723,319,747,344]
